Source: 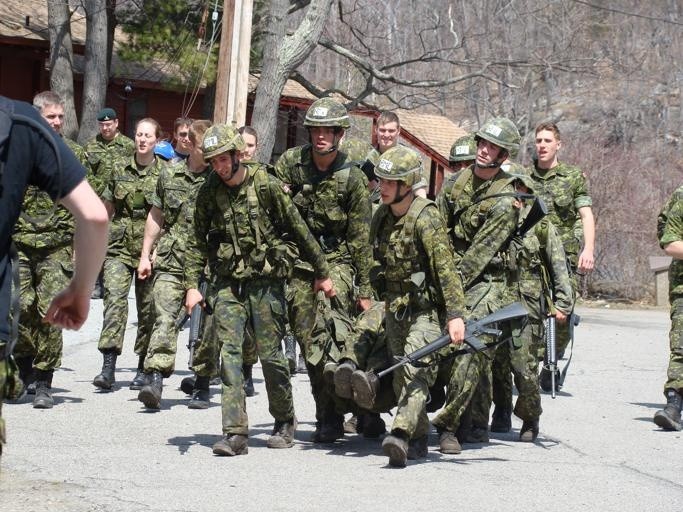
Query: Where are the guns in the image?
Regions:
[378,301,530,377]
[544,315,557,399]
[521,196,549,238]
[187,280,209,371]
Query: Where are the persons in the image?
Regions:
[659,199,683,261]
[654,187,683,430]
[192,91,595,466]
[2,93,211,412]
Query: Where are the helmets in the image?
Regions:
[202,124,247,162]
[500,162,535,194]
[477,117,520,159]
[302,97,351,128]
[449,131,477,162]
[373,145,423,187]
[336,138,382,182]
[151,141,174,160]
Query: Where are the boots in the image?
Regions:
[92,346,118,390]
[351,368,381,409]
[653,388,683,431]
[267,417,298,449]
[14,353,54,409]
[213,434,253,455]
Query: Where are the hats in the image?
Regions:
[96,107,116,120]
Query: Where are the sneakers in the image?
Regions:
[130,357,147,389]
[239,365,254,397]
[139,370,163,409]
[311,402,549,465]
[181,374,210,410]
[322,357,355,400]
